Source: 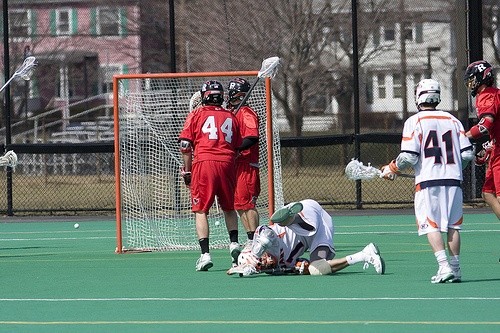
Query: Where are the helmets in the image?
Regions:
[463,59,494,98]
[238,248,267,270]
[415,78,441,107]
[201,80,225,103]
[227,77,252,103]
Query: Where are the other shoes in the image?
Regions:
[448,267,462,284]
[430,265,454,284]
[241,239,253,254]
[240,241,247,248]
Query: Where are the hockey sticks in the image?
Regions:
[345,158,415,181]
[233,57,279,116]
[0,150,17,167]
[0,55,37,93]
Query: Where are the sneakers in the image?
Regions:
[194,252,214,272]
[270,201,304,223]
[230,242,241,265]
[362,242,386,275]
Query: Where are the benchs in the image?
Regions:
[33,114,179,143]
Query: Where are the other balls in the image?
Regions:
[74,223,79,228]
[215,221,220,226]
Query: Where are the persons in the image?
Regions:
[226,199,385,277]
[178,80,242,272]
[380,77,475,283]
[465,60,500,263]
[226,77,261,241]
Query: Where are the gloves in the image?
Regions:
[474,141,493,167]
[227,255,259,276]
[379,160,402,182]
